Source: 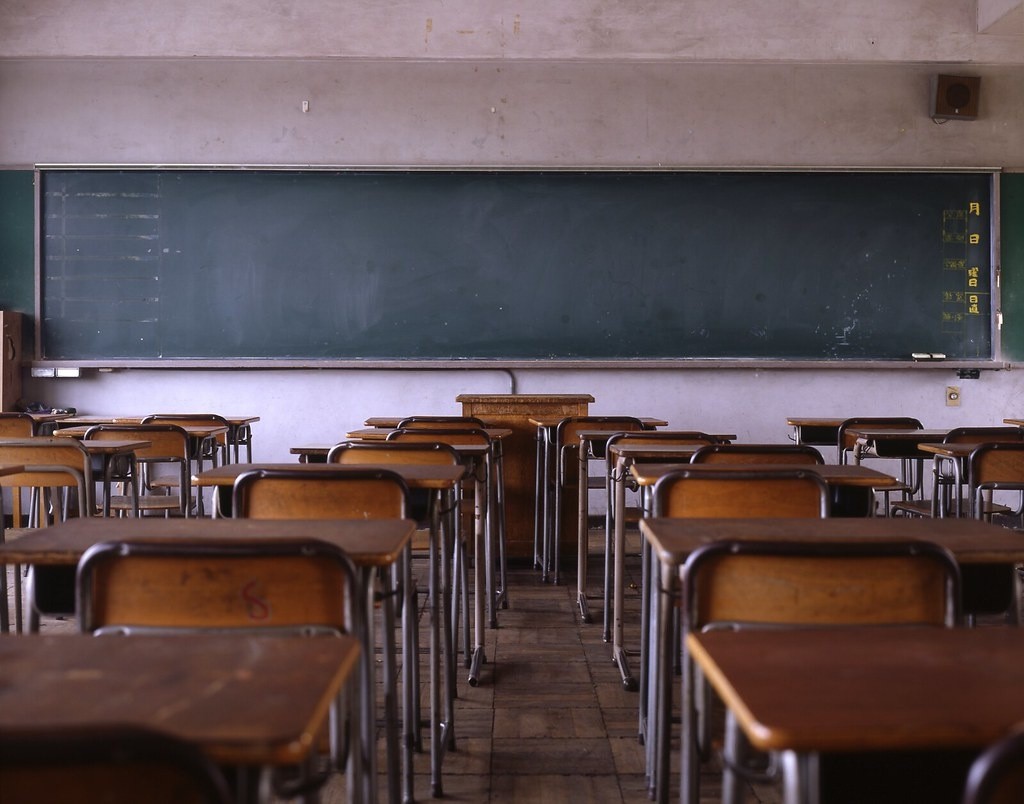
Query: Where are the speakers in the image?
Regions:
[929,73,981,121]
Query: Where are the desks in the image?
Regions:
[0,632,369,804]
[189,463,469,797]
[635,513,1024,804]
[632,460,895,739]
[286,442,493,688]
[683,621,1023,804]
[458,394,595,563]
[0,515,415,804]
[1,414,72,532]
[609,444,704,688]
[918,443,978,520]
[785,415,847,466]
[1,437,154,516]
[361,417,403,426]
[342,425,515,625]
[848,428,953,515]
[57,416,260,464]
[53,426,228,516]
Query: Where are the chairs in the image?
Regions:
[0,415,1023,804]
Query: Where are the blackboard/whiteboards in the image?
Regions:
[0,163,1024,371]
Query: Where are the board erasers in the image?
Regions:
[911,351,947,362]
